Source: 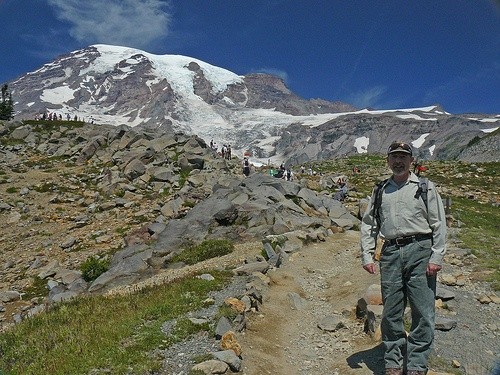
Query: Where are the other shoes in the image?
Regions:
[406,370,428,375]
[383,368,404,375]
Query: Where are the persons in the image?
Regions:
[210,139,361,182]
[35,112,95,124]
[361,143,448,375]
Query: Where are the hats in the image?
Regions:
[387,140,412,155]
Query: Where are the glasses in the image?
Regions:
[387,143,411,151]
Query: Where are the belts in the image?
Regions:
[385,233,432,246]
